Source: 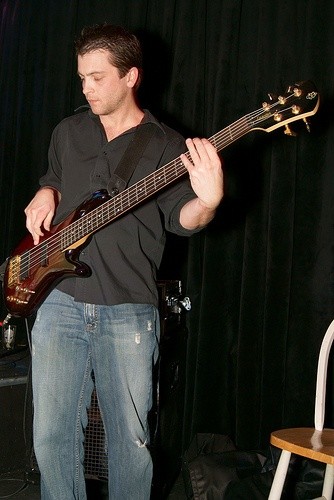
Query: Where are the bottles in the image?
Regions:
[2,315,17,354]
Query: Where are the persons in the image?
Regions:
[24,22,224,500]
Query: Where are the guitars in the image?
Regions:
[3,84,318,317]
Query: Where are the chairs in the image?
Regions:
[264,320,334,500]
